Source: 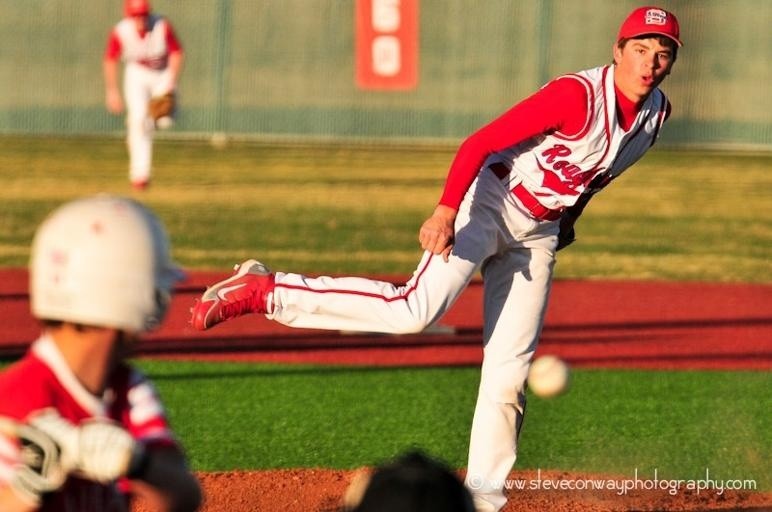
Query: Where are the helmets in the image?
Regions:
[28,193,187,333]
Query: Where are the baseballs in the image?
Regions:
[527,355,571,399]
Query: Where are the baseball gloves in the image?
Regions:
[149,94,175,118]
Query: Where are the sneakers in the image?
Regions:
[189,254,276,331]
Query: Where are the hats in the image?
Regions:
[125,0,152,18]
[615,5,682,48]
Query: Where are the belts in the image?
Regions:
[489,162,561,222]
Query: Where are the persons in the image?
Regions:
[340,448,476,512]
[1,192,205,511]
[186,6,684,511]
[103,1,183,190]
